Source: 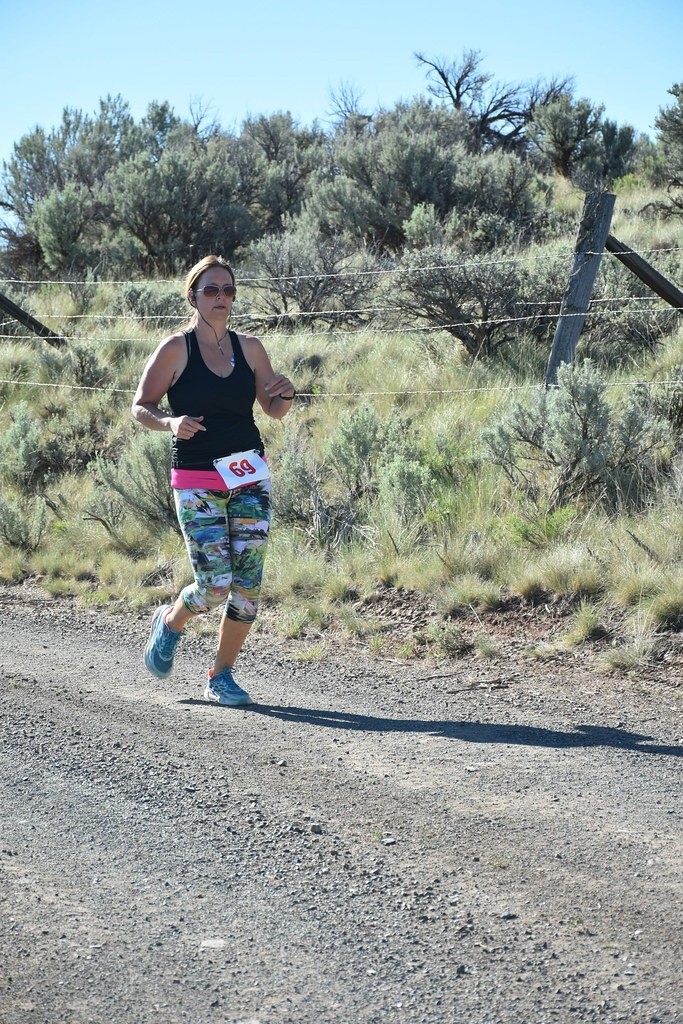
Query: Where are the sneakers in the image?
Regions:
[204,666,252,705]
[144,604,186,679]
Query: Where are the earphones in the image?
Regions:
[191,295,197,301]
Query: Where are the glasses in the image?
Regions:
[195,285,237,298]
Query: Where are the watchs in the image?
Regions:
[279,390,295,400]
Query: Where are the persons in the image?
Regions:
[130,256,297,705]
